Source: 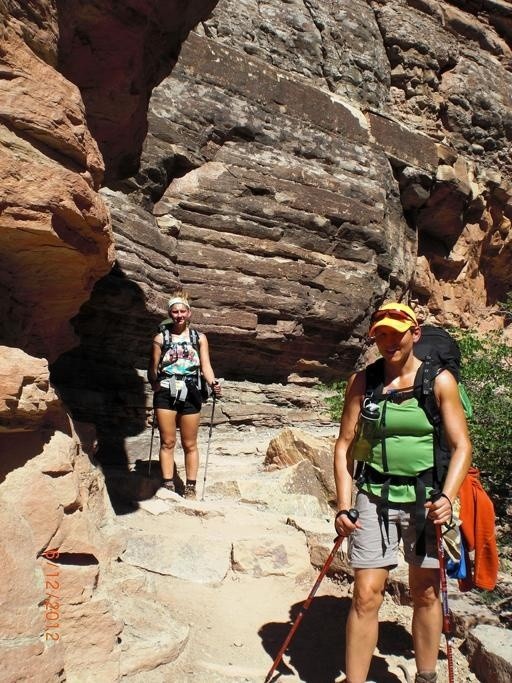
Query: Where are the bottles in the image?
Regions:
[349,401,380,463]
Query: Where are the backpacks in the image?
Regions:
[159,318,200,381]
[352,325,461,487]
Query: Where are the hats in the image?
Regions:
[168,298,191,310]
[369,304,417,338]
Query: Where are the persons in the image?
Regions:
[146,296,223,499]
[333,301,473,682]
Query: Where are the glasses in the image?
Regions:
[371,308,413,324]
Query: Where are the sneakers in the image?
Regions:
[185,484,197,500]
[163,480,175,493]
